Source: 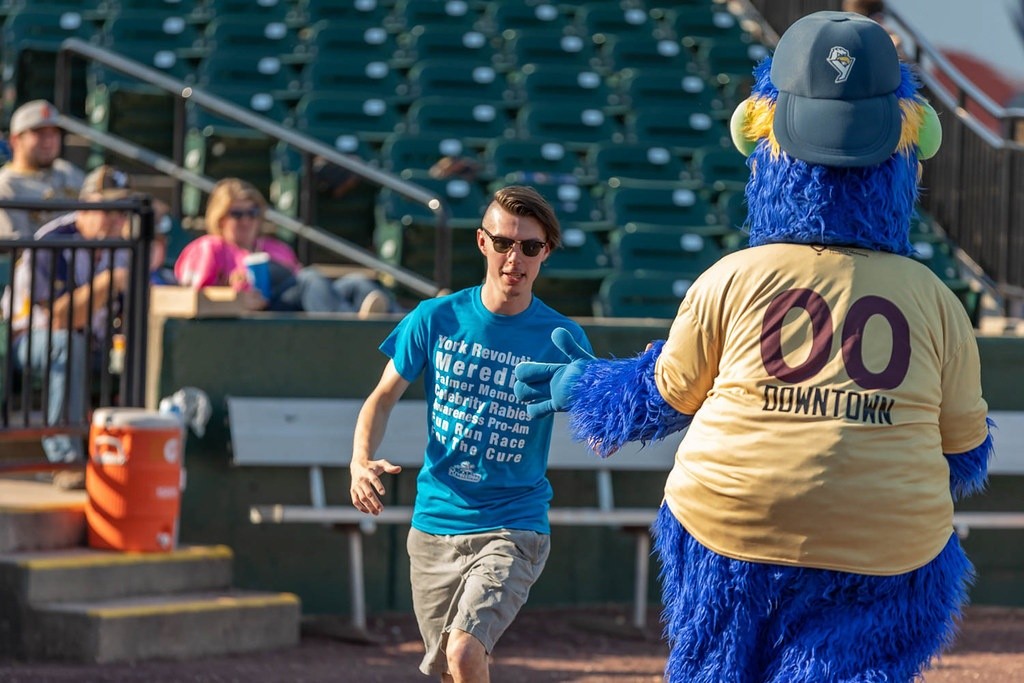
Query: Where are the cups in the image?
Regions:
[242,251,271,302]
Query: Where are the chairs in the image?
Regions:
[0,0,980,406]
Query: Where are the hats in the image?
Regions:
[80,167,129,194]
[11,100,63,137]
[770,11,902,166]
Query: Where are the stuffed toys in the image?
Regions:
[514,9,993,682]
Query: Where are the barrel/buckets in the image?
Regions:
[83,405,182,554]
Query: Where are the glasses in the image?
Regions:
[229,206,261,221]
[482,226,550,257]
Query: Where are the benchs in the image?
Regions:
[217,390,1024,642]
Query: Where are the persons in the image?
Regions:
[0,98,404,491]
[350,187,592,683]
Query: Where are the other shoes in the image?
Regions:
[43,434,78,462]
